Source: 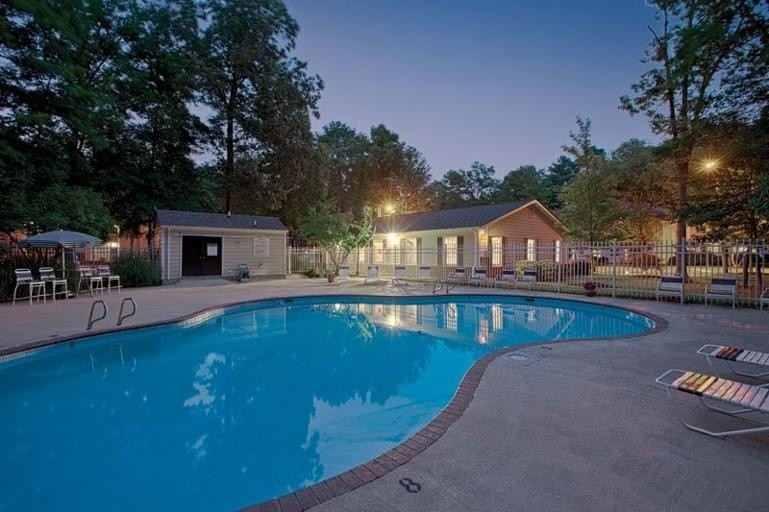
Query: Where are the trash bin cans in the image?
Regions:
[238,264,250,282]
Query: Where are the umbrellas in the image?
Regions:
[16,227,104,281]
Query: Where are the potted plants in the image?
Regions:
[581,279,596,296]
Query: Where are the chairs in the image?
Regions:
[655,272,769,440]
[334,266,538,295]
[12,264,123,307]
